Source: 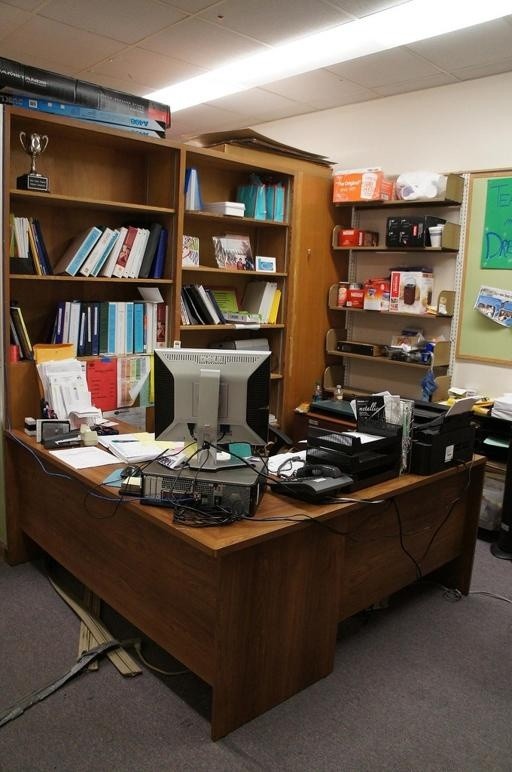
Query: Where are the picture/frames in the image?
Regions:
[454,169,512,369]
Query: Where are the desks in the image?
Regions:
[11,430,489,742]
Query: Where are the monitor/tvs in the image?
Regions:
[154,348,272,472]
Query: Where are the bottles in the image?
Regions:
[334,384,343,400]
[312,380,322,402]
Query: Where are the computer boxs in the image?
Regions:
[140,448,268,517]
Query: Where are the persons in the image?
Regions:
[243,257,254,271]
[236,258,245,271]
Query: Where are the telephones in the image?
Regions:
[268,465,354,503]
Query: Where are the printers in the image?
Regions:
[402,410,477,476]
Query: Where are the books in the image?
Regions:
[95,431,170,466]
[7,207,172,424]
[350,390,413,472]
[179,271,288,338]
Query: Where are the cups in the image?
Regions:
[429,227,442,247]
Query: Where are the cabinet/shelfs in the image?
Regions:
[3,106,332,440]
[322,173,463,413]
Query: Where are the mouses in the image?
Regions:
[120,464,142,479]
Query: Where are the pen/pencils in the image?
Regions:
[112,439,140,443]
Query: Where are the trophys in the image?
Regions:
[15,131,51,193]
